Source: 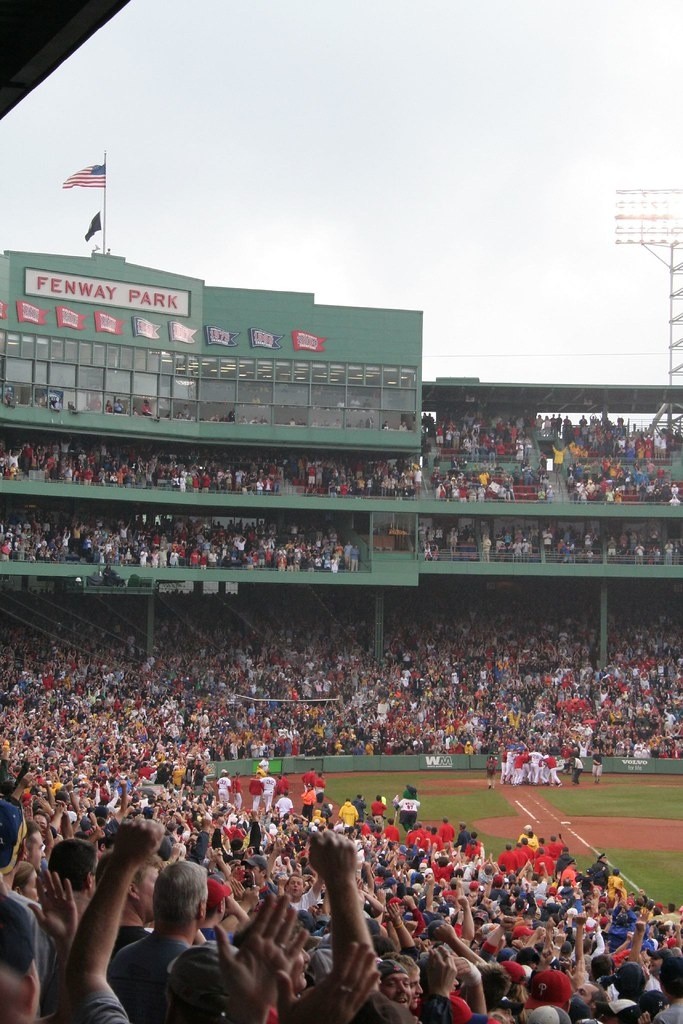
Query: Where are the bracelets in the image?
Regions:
[392,921,403,929]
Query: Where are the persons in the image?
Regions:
[0,381,683,1023]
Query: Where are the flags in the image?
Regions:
[62,163,105,188]
[85,211,102,242]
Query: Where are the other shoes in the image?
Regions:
[500,777,562,787]
[489,785,491,789]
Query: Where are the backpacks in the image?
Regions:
[487,761,494,772]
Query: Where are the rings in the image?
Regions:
[337,984,354,994]
[275,941,290,952]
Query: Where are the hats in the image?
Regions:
[525,969,572,1009]
[307,946,333,984]
[378,960,408,979]
[384,853,619,961]
[449,995,488,1024]
[595,999,640,1024]
[647,949,673,957]
[529,1005,572,1024]
[0,794,26,874]
[495,997,524,1015]
[524,824,532,835]
[0,894,35,972]
[614,962,640,991]
[241,856,267,869]
[501,961,526,984]
[459,822,466,828]
[207,878,231,908]
[167,941,240,1011]
